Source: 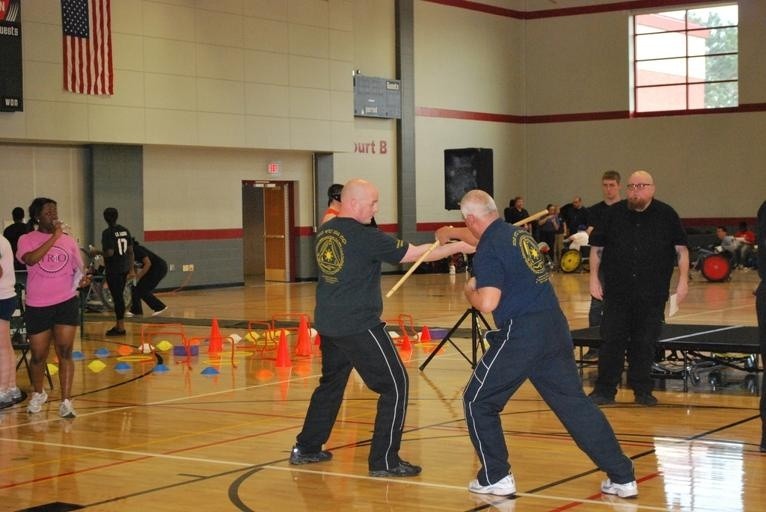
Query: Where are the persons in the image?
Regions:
[568,227,593,252]
[3,208,35,270]
[16,198,90,417]
[588,169,688,407]
[504,199,514,222]
[435,190,639,499]
[689,226,736,280]
[290,178,477,478]
[124,237,169,316]
[753,198,765,454]
[321,183,344,223]
[507,197,537,232]
[101,207,133,336]
[586,171,625,233]
[559,197,585,237]
[537,204,560,262]
[734,221,755,270]
[0,234,22,405]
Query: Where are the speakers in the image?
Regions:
[445,148,492,210]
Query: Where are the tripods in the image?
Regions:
[419,209,492,371]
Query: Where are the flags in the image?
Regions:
[61,0,114,94]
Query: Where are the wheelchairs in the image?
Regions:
[558,246,590,273]
[696,242,751,284]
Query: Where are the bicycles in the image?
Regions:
[78,246,132,309]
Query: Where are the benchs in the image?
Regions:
[566,320,765,390]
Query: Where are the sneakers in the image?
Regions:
[152,306,167,316]
[59,399,77,418]
[588,393,615,406]
[124,312,142,318]
[635,395,658,406]
[369,461,422,477]
[106,327,125,336]
[0,386,23,409]
[601,477,639,497]
[27,389,49,413]
[289,446,332,465]
[469,472,516,496]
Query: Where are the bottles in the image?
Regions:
[88,244,101,260]
[449,274,456,284]
[53,219,72,234]
[449,264,455,276]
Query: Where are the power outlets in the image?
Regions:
[182,264,194,272]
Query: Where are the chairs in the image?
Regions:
[8,282,54,390]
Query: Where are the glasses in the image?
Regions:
[627,184,651,190]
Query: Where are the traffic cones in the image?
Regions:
[399,335,412,352]
[294,357,312,389]
[201,318,225,353]
[418,324,432,343]
[292,314,313,358]
[274,365,292,402]
[272,328,293,366]
[207,354,221,384]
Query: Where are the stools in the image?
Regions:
[85,275,106,300]
